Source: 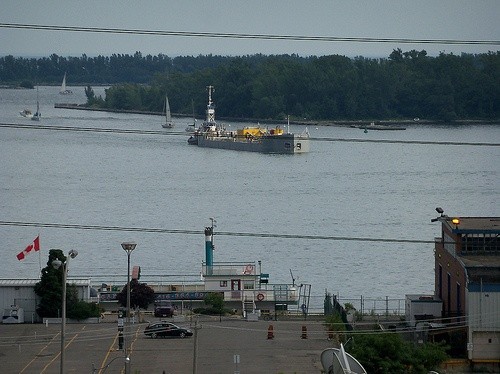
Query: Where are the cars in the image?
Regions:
[143,322,194,340]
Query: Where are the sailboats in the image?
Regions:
[31,64,43,121]
[59,72,72,95]
[161,93,176,128]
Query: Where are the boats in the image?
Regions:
[185,118,204,132]
[188,83,311,155]
[19,108,33,119]
[87,217,302,312]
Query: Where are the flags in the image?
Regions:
[16,236,39,261]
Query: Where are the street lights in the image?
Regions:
[120,240,138,326]
[51,249,78,374]
